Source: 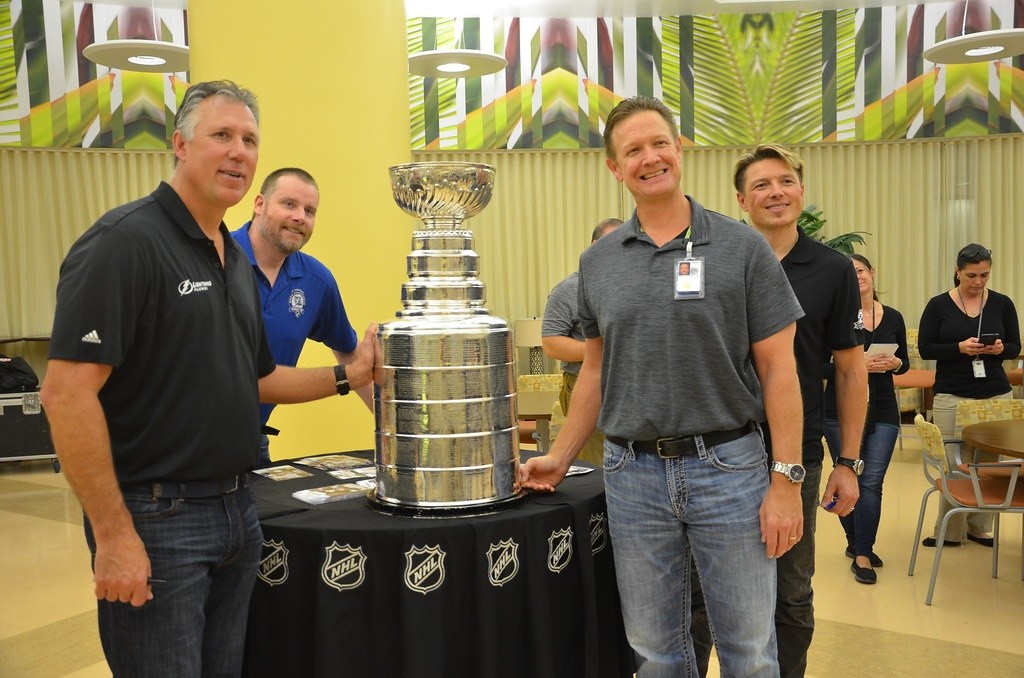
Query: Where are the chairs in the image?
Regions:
[513,318,565,460]
[907,399,1024,606]
[1002,355,1024,395]
[891,329,935,452]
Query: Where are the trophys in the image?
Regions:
[363,160,530,518]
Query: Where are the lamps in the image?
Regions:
[920,0,1024,63]
[82,1,189,72]
[407,19,507,79]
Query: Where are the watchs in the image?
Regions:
[832,456,864,476]
[770,461,807,483]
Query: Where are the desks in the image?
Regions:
[242,453,638,678]
[962,420,1024,581]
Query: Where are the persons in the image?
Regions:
[542,218,625,467]
[918,243,1022,547]
[39,80,375,678]
[690,143,871,678]
[230,167,375,464]
[679,262,690,275]
[822,255,910,585]
[519,95,804,678]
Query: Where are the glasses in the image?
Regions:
[957,249,992,264]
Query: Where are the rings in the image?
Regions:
[789,535,797,540]
[849,508,854,511]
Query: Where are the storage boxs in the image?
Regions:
[0,392,62,474]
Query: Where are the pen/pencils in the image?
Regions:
[824,501,836,510]
[147,578,166,583]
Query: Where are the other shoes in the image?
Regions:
[851,559,877,584]
[846,547,882,567]
[922,537,961,547]
[966,532,994,547]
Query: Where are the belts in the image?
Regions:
[606,421,755,459]
[120,474,249,498]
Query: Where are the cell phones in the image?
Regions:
[977,334,1000,348]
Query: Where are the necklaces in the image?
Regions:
[862,309,873,317]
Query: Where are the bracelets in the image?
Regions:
[335,365,351,397]
